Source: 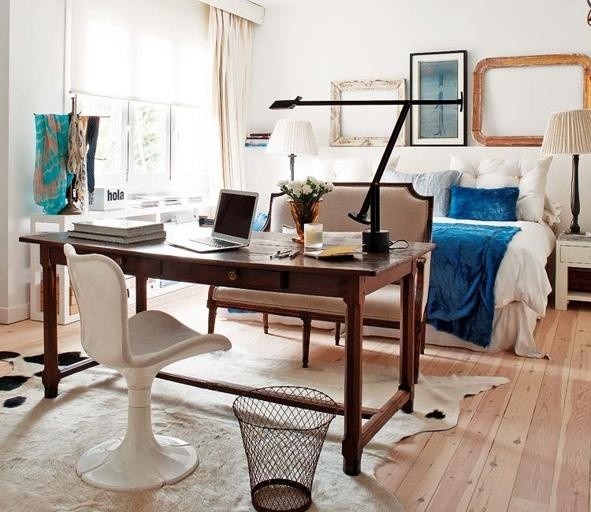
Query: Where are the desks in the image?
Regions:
[17,223,437,476]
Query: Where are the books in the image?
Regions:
[67,229,166,245]
[243,132,272,148]
[73,218,164,239]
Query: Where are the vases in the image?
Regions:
[285,198,324,244]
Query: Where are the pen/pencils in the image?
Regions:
[269,248,301,259]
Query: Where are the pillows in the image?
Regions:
[306,155,553,228]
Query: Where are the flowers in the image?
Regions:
[274,176,334,229]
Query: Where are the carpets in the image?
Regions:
[0,349,511,512]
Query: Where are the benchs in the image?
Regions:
[206,182,434,385]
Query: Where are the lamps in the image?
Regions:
[540,110,591,236]
[265,119,317,181]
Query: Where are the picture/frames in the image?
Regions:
[410,50,467,147]
[329,79,408,148]
[471,54,591,147]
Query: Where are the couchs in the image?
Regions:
[216,194,562,360]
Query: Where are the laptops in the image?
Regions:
[168,188,260,253]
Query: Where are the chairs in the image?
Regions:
[62,242,231,495]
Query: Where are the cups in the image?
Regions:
[303,222,323,255]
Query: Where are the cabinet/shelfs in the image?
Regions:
[29,195,206,325]
[554,229,591,311]
